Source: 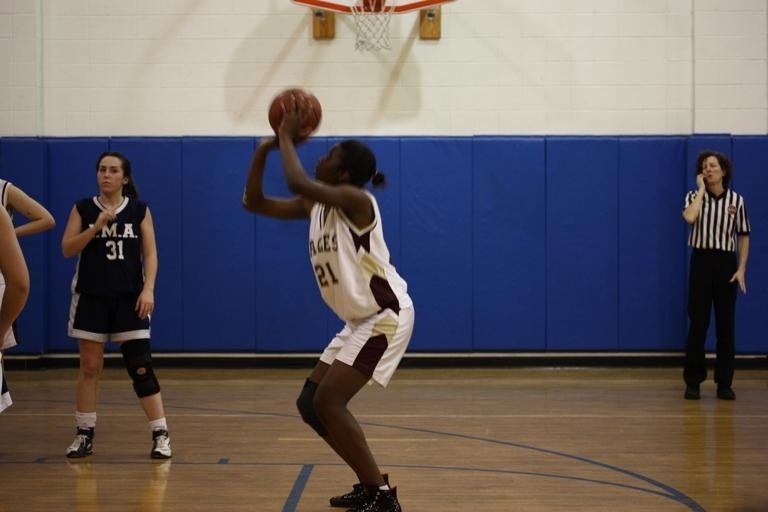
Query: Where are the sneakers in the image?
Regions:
[330,473,401,512]
[151,429,172,459]
[684,384,700,399]
[65,426,94,458]
[717,383,735,400]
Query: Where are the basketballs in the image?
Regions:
[268,90,322,139]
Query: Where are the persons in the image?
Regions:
[65,151,173,457]
[681,148,751,399]
[241,90,415,512]
[0,203,30,418]
[0,177,57,353]
